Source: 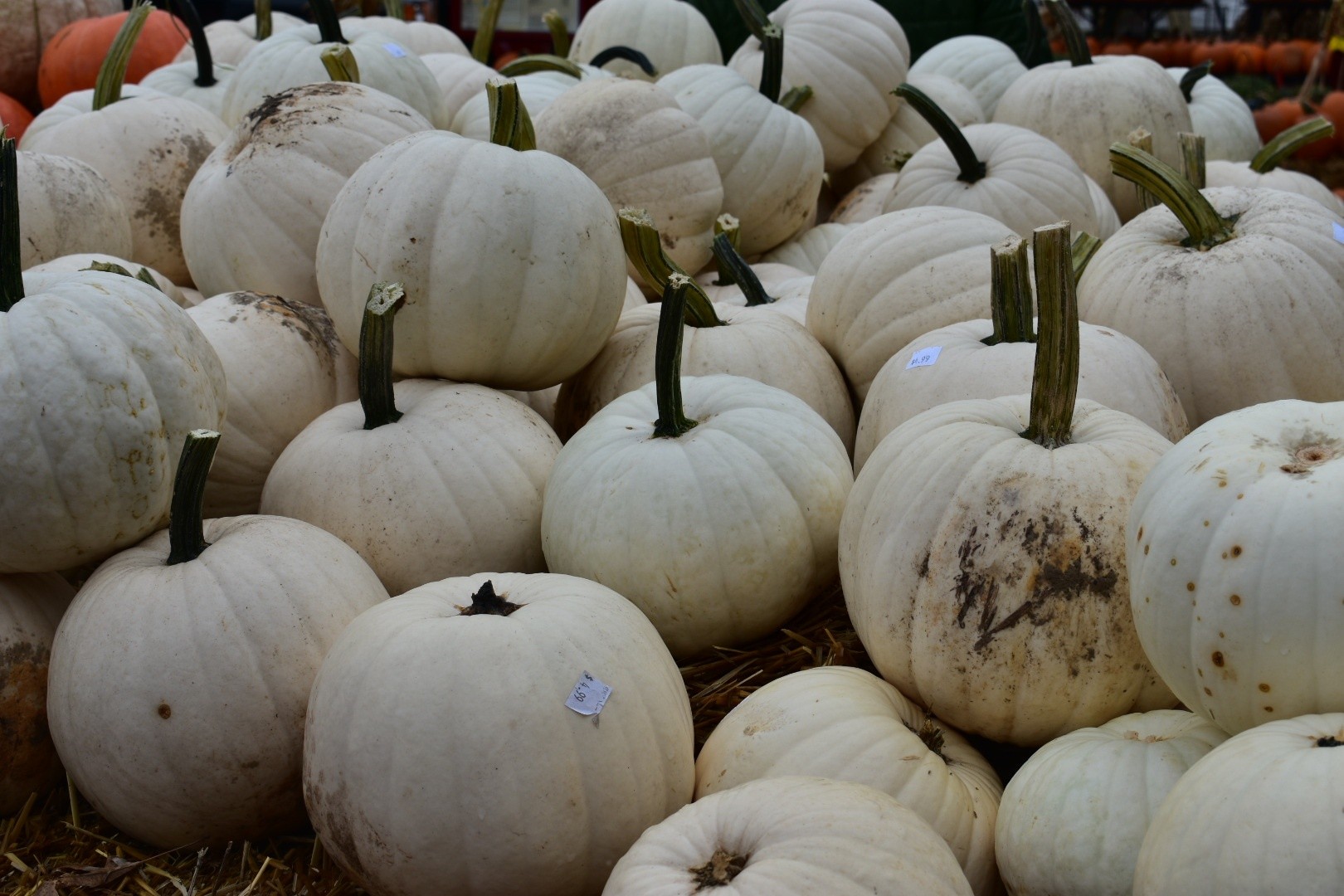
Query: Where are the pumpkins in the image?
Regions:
[0,0,1344,896]
